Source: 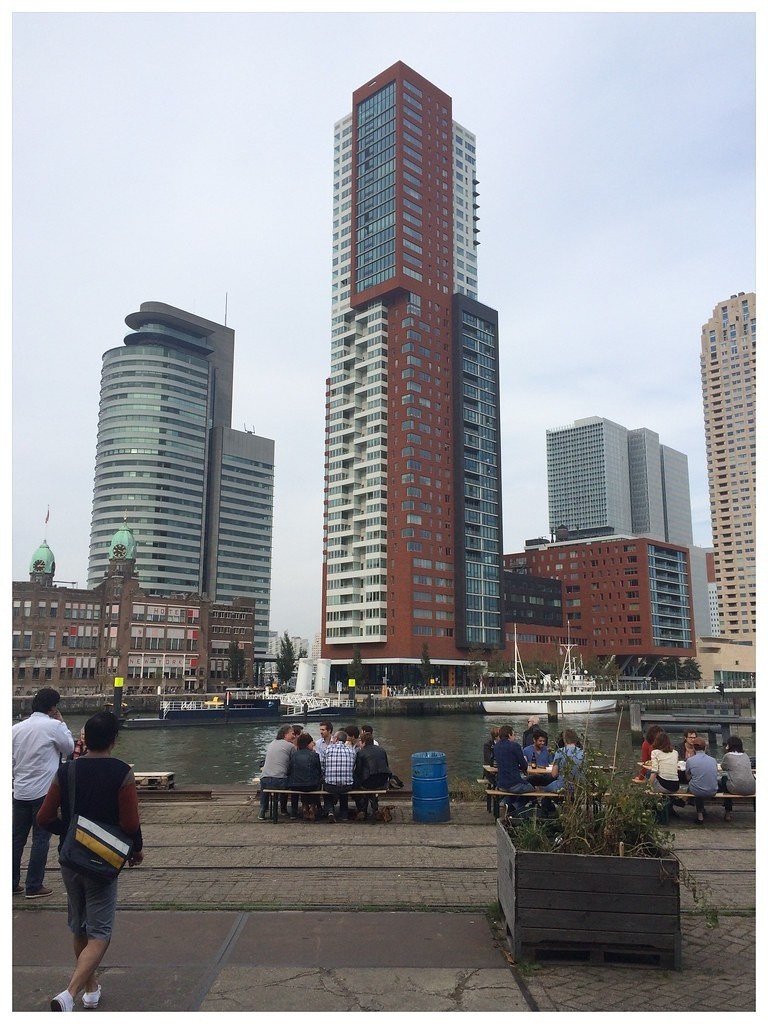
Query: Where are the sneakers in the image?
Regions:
[13,886,24,894]
[25,886,53,898]
[82,985,101,1009]
[50,989,74,1012]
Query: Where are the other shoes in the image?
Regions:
[504,803,512,817]
[694,818,704,824]
[673,798,686,807]
[257,802,378,822]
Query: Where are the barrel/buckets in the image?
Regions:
[411,751,450,823]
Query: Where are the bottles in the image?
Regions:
[531,752,537,769]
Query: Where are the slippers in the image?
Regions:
[724,816,732,821]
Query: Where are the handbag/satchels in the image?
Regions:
[59,814,133,884]
[389,776,404,790]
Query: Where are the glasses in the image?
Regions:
[687,737,696,739]
[528,721,533,723]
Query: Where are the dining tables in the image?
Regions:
[481,762,729,772]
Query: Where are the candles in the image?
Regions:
[32,560,45,573]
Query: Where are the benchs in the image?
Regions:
[263,789,386,825]
[477,777,756,822]
[134,770,176,791]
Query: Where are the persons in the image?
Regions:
[483,716,593,819]
[635,726,756,824]
[12,689,74,898]
[247,683,283,694]
[387,685,421,697]
[66,726,88,760]
[36,712,144,1011]
[258,721,389,820]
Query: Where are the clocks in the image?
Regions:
[113,545,127,559]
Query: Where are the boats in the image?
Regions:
[481,620,618,715]
